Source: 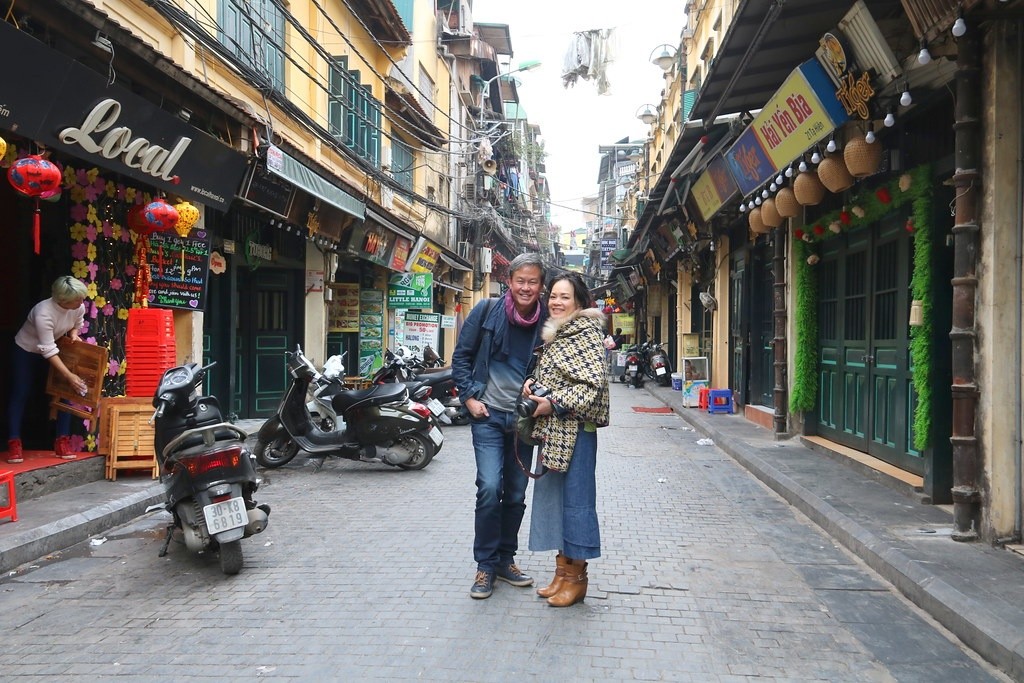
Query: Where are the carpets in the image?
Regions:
[631,407,673,413]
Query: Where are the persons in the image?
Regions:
[519,272,609,607]
[453,254,549,599]
[610,328,623,348]
[6,275,88,464]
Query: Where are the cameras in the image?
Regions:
[517,381,551,418]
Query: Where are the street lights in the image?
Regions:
[474,60,542,307]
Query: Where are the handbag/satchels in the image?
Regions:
[515,414,539,447]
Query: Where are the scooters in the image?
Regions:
[254,342,444,469]
[148,359,271,575]
[307,343,473,457]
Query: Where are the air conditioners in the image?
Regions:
[458,242,473,261]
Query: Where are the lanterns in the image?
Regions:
[7,154,61,256]
[127,197,199,281]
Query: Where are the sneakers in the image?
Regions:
[470,569,496,598]
[55,435,76,460]
[7,439,23,463]
[495,563,533,586]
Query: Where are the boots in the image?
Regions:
[539,554,573,597]
[547,560,589,607]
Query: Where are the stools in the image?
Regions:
[0,469,18,522]
[125,308,176,397]
[699,388,723,410]
[708,389,733,415]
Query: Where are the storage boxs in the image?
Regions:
[671,373,682,390]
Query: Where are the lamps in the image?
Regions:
[614,195,628,207]
[91,30,112,53]
[618,174,640,188]
[176,107,192,123]
[649,43,681,70]
[636,103,660,124]
[625,145,644,162]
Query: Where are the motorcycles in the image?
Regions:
[621,338,673,388]
